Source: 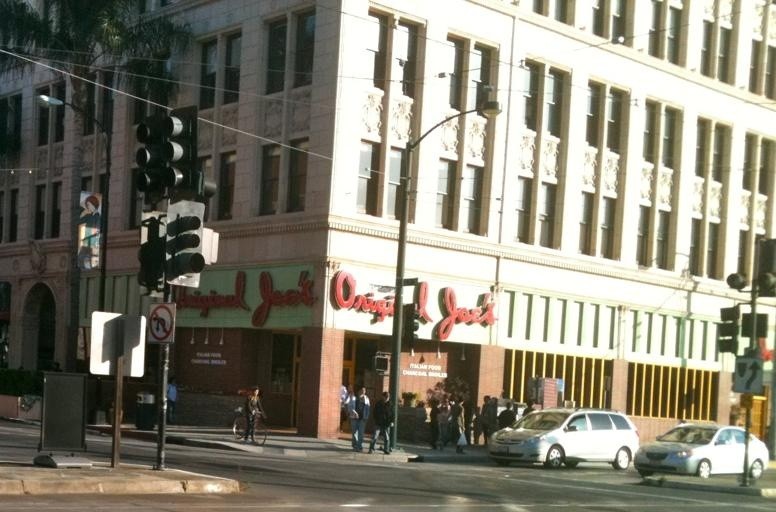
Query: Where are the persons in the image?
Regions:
[340,385,348,432]
[78,195,101,269]
[427,392,536,455]
[166,375,177,420]
[245,386,264,441]
[348,385,371,452]
[369,390,393,454]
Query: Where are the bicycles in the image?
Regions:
[233,407,268,447]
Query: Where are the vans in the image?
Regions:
[491,409,642,470]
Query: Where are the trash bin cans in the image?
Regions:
[135,391,155,431]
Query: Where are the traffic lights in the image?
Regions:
[163,200,206,289]
[163,103,204,198]
[203,177,217,201]
[134,113,166,202]
[717,305,740,354]
[402,301,422,347]
[376,357,387,371]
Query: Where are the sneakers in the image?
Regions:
[353,447,392,454]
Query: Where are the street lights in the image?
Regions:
[726,271,776,486]
[36,93,113,419]
[383,102,504,447]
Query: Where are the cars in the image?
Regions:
[631,422,770,485]
